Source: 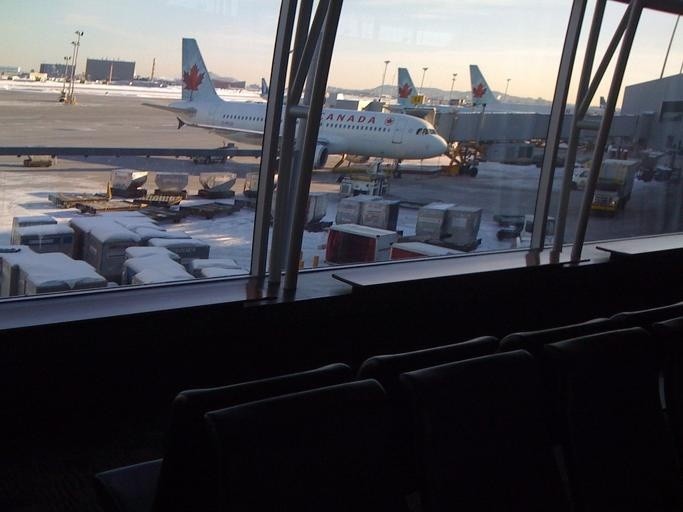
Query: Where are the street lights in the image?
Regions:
[59,30,84,104]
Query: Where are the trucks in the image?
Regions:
[590,158,637,216]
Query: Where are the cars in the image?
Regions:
[570,168,590,189]
